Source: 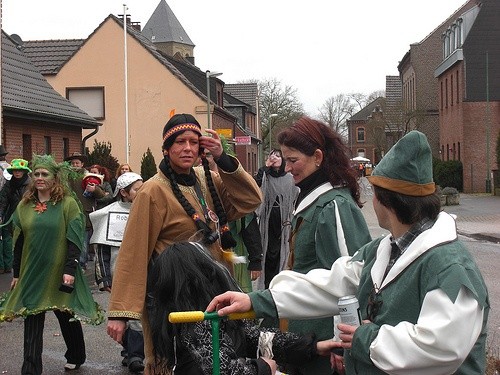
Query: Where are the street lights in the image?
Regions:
[269,114,279,152]
[206,70,223,138]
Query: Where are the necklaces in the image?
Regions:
[175,169,210,223]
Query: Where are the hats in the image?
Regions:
[112,172,144,198]
[162,114,204,144]
[64,152,88,164]
[7,159,32,173]
[81,176,102,189]
[366,131,435,196]
[0,144,10,155]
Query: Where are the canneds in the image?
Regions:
[338,295,363,327]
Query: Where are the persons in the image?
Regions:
[207,130,491,375]
[107,114,264,375]
[253,148,300,291]
[89,172,146,373]
[278,118,372,375]
[352,163,366,177]
[0,154,105,375]
[0,152,133,292]
[145,242,343,375]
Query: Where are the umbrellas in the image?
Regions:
[350,156,370,162]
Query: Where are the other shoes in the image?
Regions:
[64,364,80,371]
[130,362,144,371]
